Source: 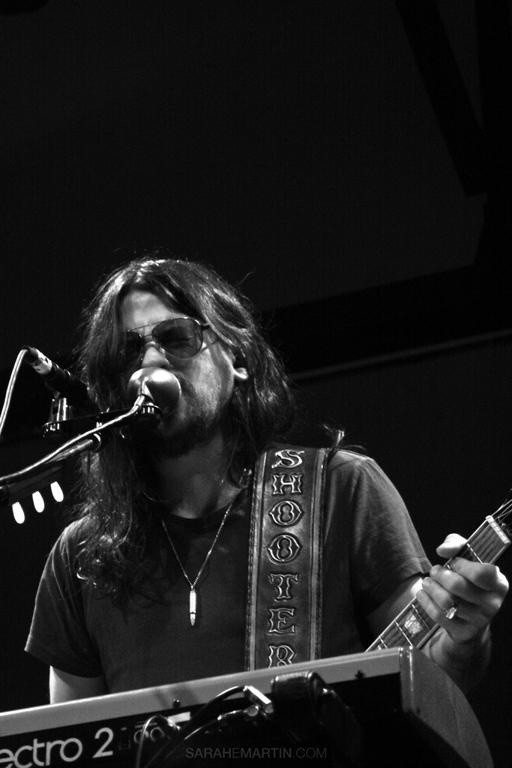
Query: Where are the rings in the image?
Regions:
[445,606,460,622]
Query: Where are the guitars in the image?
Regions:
[362,499,512,652]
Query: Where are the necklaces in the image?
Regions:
[159,466,250,632]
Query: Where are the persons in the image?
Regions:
[23,252,509,720]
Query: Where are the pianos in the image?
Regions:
[0,645,493,768]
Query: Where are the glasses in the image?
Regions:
[97,317,210,380]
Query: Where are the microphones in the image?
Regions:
[24,344,103,414]
[123,367,183,438]
